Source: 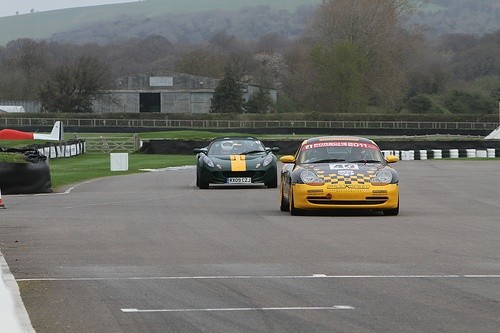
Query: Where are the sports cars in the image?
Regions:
[281,136,400,214]
[193,136,279,189]
